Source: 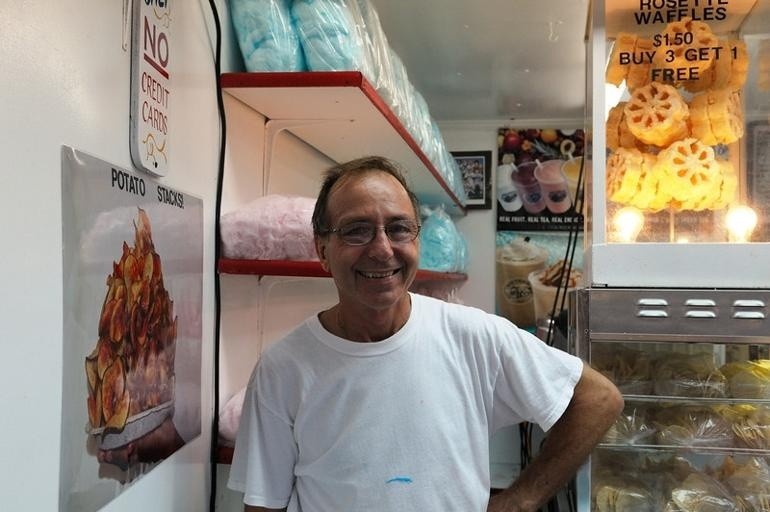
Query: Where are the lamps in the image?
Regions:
[612,205,645,243]
[724,204,757,243]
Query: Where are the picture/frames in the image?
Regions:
[448,149,492,210]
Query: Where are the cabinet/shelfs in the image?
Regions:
[562,289,770,511]
[213,68,471,466]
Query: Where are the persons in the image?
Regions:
[224,154,624,511]
[456,158,484,196]
[96,415,185,470]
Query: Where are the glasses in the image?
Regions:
[324,218,421,246]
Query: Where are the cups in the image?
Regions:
[495,164,523,212]
[528,269,586,323]
[560,156,585,215]
[509,162,545,215]
[497,246,549,328]
[533,159,571,214]
